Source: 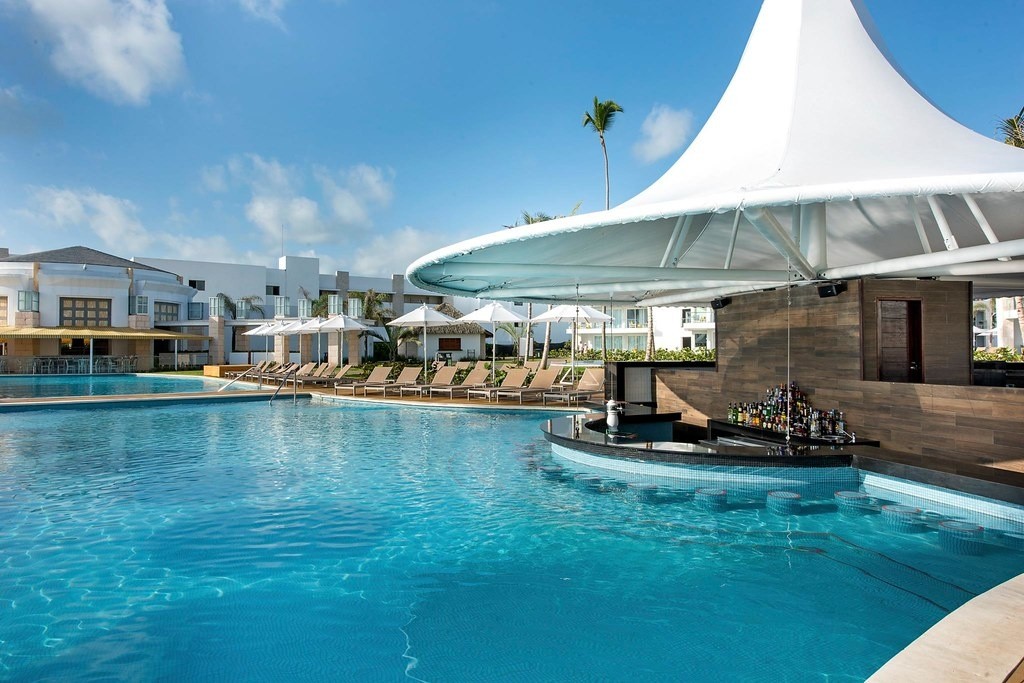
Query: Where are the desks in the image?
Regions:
[707,418,880,447]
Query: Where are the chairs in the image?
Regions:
[0,354,139,375]
[224,358,604,406]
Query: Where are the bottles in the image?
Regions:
[727,380,845,437]
[766,445,844,456]
